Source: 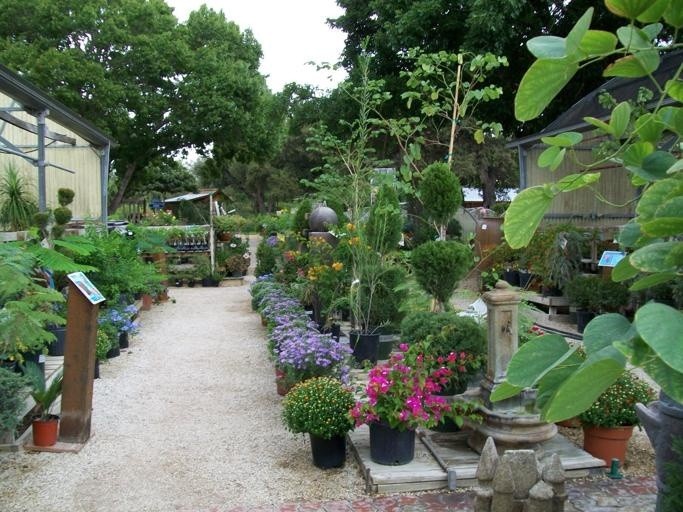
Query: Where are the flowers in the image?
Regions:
[278,375,358,439]
[573,368,657,430]
[248,209,353,375]
[94,292,150,360]
[351,342,467,428]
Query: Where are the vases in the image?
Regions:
[579,423,638,471]
[44,328,65,356]
[366,418,419,468]
[94,358,102,380]
[301,432,350,469]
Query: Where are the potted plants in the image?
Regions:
[348,208,399,370]
[23,360,62,448]
[492,220,656,336]
[404,312,486,436]
[124,192,252,288]
